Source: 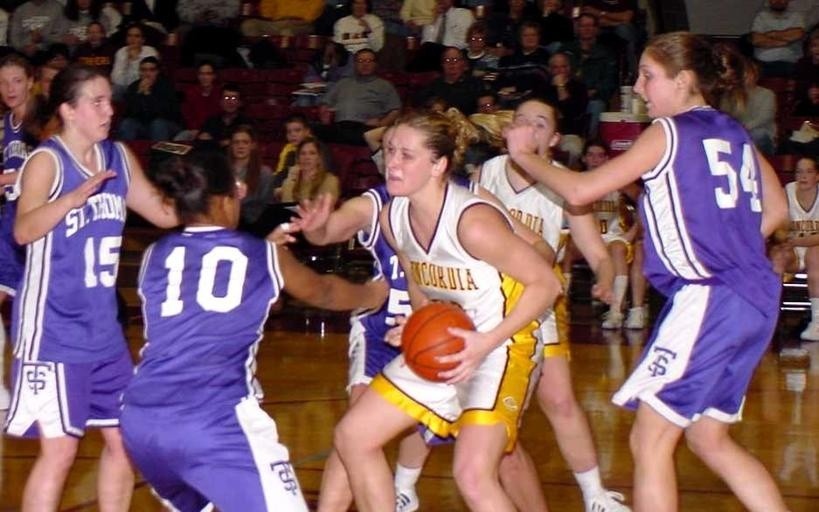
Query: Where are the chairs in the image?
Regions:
[130,142,151,169]
[763,78,798,184]
[221,34,324,118]
[385,33,438,104]
[335,145,384,192]
[259,140,284,168]
[170,68,198,95]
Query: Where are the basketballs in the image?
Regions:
[401,302,477,382]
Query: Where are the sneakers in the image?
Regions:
[799,320,819,341]
[624,307,645,330]
[585,489,632,512]
[601,312,625,330]
[395,487,419,512]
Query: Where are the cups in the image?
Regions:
[307,35,320,51]
[618,85,646,117]
[408,37,416,52]
